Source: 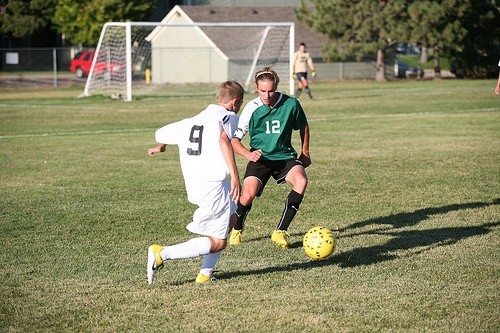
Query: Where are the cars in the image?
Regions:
[395,59,425,79]
[69,51,135,82]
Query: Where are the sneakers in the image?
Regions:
[196,273,218,284]
[271,229,289,247]
[147,245,164,284]
[230,227,242,244]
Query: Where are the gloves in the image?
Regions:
[312,70,316,77]
[293,73,297,79]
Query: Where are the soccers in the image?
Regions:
[302,227,336,258]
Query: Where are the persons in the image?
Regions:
[147,81,245,286]
[292,42,317,101]
[229,69,312,248]
